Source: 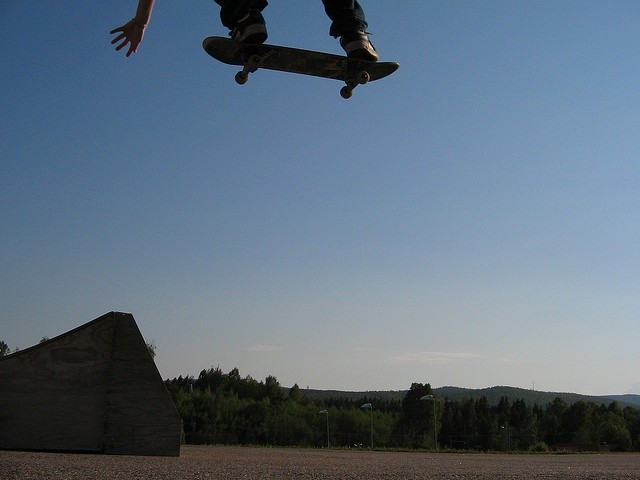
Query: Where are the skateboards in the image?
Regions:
[202,36,399,99]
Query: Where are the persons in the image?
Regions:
[110,0,400,100]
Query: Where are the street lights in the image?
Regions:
[360,403,373,449]
[419,395,437,451]
[319,410,330,449]
[500,426,510,452]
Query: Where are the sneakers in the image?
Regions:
[228,11,268,43]
[339,29,379,62]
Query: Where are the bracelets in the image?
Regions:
[132,18,146,29]
